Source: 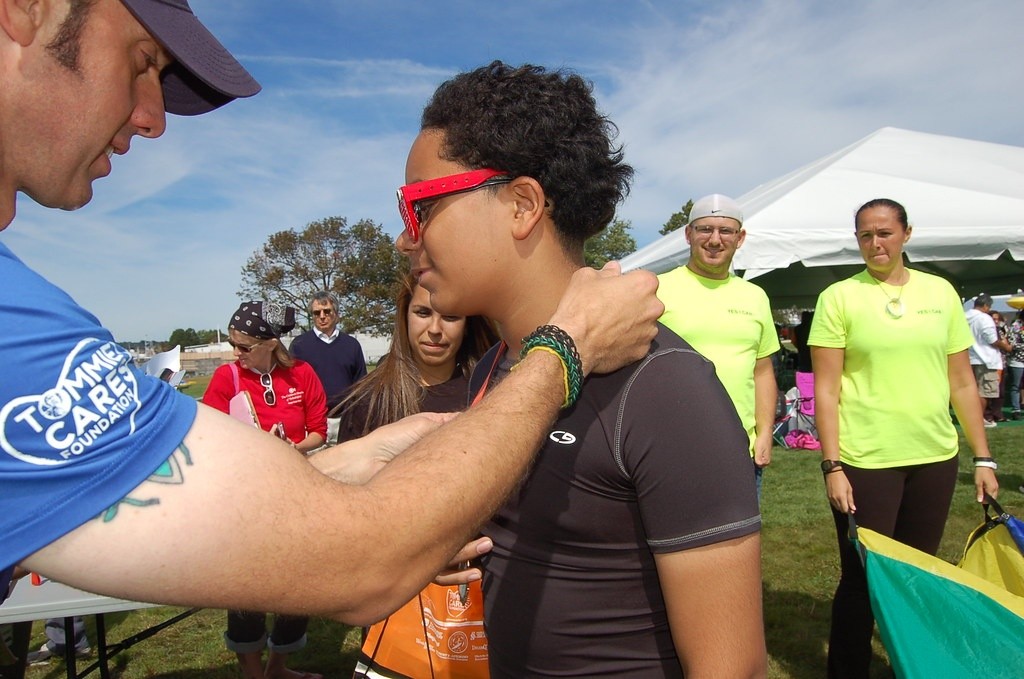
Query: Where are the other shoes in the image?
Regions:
[265,672,322,679]
[40,643,92,660]
[983,411,1021,428]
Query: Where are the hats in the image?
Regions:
[119,0,263,117]
[688,194,743,227]
[154,368,186,390]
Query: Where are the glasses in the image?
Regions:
[309,308,334,317]
[689,225,739,239]
[397,168,549,243]
[227,336,261,353]
[260,372,276,406]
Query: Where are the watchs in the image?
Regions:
[820,459,843,471]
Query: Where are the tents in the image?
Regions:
[614,127,1024,351]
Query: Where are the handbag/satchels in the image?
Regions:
[352,572,490,679]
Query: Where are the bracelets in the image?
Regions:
[824,468,844,475]
[972,457,997,470]
[510,325,585,409]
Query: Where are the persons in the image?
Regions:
[330,268,499,679]
[27,615,93,665]
[201,300,328,679]
[396,61,768,679]
[777,288,1024,428]
[655,194,780,509]
[288,292,366,457]
[806,200,999,679]
[0,0,666,679]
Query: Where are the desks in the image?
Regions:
[0,572,169,679]
